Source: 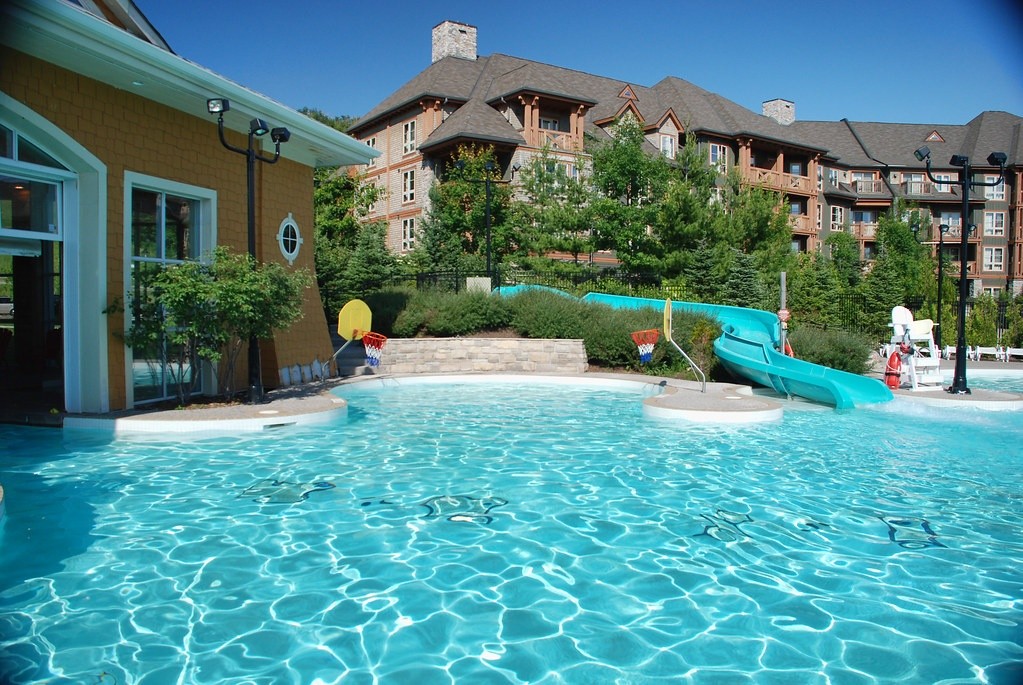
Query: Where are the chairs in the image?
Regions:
[888,305,946,392]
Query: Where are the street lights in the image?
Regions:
[208,97,294,405]
[454,161,521,280]
[911,146,1008,396]
[911,222,979,357]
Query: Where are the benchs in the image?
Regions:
[879,344,1023,362]
